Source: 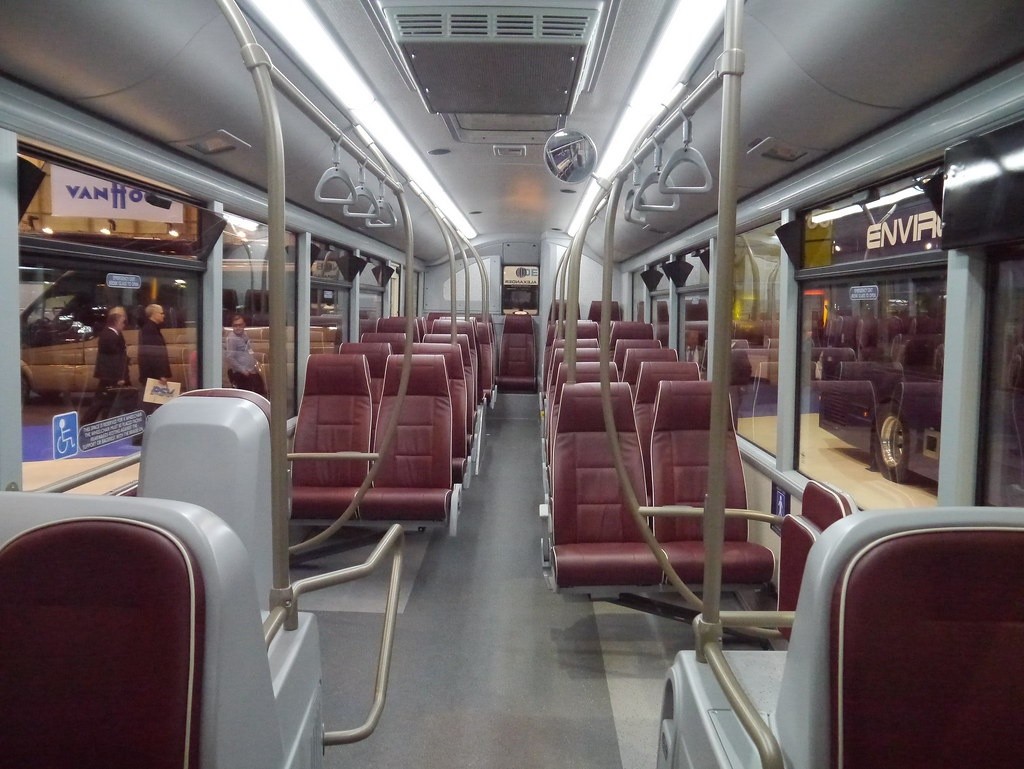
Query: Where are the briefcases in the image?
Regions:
[104,384,139,410]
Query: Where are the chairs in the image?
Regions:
[541,381,665,587]
[0,514,203,769]
[646,377,777,587]
[688,290,946,494]
[289,295,678,541]
[637,299,669,350]
[829,523,1024,769]
[628,351,703,527]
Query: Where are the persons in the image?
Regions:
[132,304,172,412]
[226,316,264,396]
[685,330,705,368]
[80,306,137,427]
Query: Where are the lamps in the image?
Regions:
[235,0,480,249]
[812,180,926,231]
[564,0,734,241]
